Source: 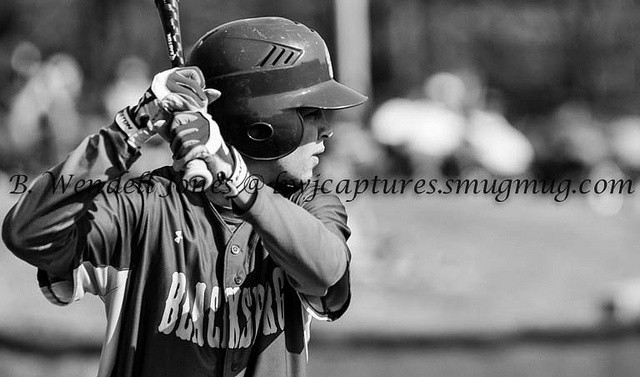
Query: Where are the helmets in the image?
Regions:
[188,16,369,162]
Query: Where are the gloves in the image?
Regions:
[154,109,247,199]
[114,65,222,150]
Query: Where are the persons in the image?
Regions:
[1,18,369,377]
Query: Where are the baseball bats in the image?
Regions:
[153,0,214,193]
